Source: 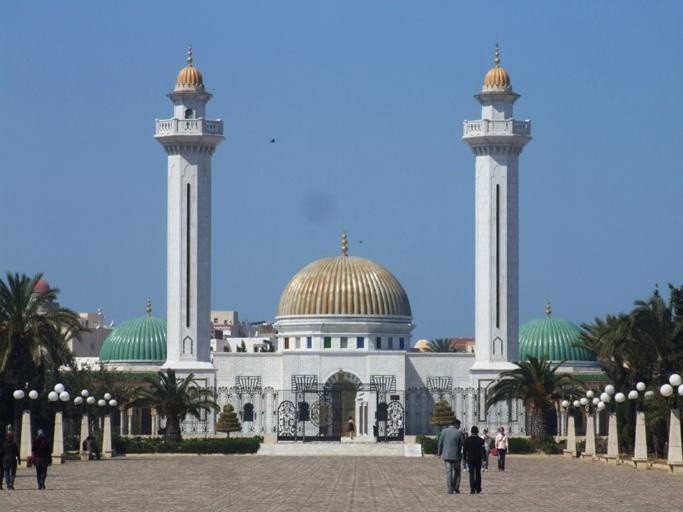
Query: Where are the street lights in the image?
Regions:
[660,373,683,463]
[98,393,118,452]
[74,389,96,453]
[48,383,71,455]
[12,381,39,456]
[579,384,625,456]
[562,399,581,452]
[628,382,654,459]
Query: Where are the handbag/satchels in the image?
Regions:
[492,448,498,456]
[30,456,36,463]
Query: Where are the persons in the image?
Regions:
[462,433,472,472]
[347,416,354,440]
[436,419,464,494]
[3,433,19,490]
[495,427,508,472]
[86,436,98,460]
[467,425,486,496]
[33,428,50,489]
[479,428,491,472]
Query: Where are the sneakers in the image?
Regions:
[38,484,45,489]
[464,467,469,471]
[0,484,14,490]
[481,468,488,472]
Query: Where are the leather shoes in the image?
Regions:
[447,490,460,494]
[471,488,481,494]
